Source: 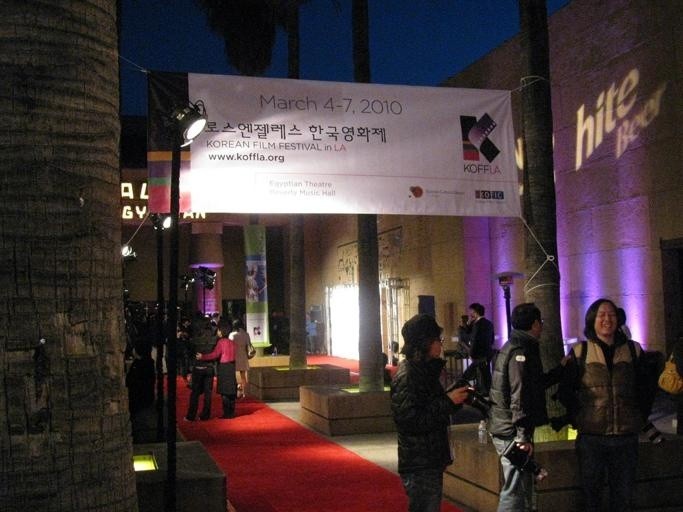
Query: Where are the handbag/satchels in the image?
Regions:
[246,342,256,360]
[658,347,682,395]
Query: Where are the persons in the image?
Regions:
[458,303,479,385]
[246,265,266,301]
[615,306,632,341]
[125,336,156,444]
[381,351,392,388]
[389,313,469,511]
[468,305,494,396]
[484,302,571,511]
[557,298,649,512]
[269,312,328,357]
[124,300,251,422]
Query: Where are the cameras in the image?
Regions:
[444,376,498,419]
[505,441,549,484]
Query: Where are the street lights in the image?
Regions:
[119,242,136,265]
[156,95,210,509]
[146,211,174,440]
[178,263,216,317]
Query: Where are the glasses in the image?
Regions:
[432,337,446,344]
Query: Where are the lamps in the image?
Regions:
[149,209,175,237]
[167,93,210,146]
[176,263,218,295]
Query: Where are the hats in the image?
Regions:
[401,313,443,346]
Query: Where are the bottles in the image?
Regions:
[479,419,487,444]
